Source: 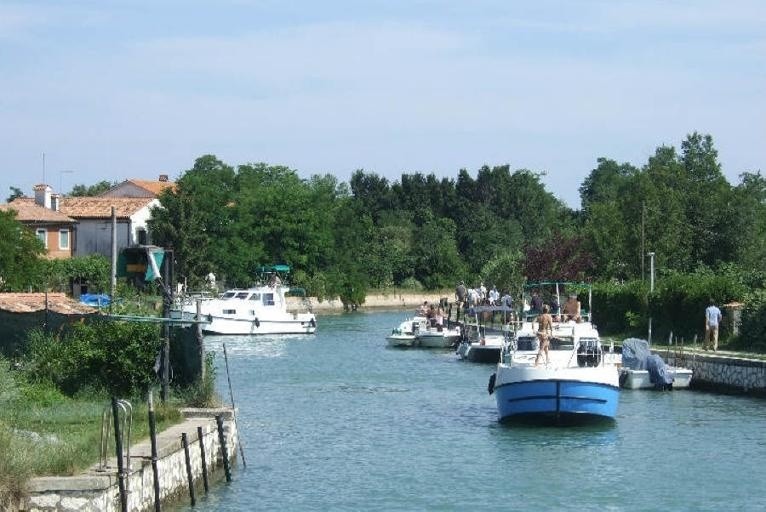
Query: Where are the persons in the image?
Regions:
[532,304,555,367]
[203,269,215,289]
[701,298,723,352]
[267,272,282,291]
[420,280,584,334]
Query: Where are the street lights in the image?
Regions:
[647,251,655,345]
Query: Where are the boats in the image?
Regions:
[384,316,461,348]
[169,264,318,336]
[456,283,694,424]
